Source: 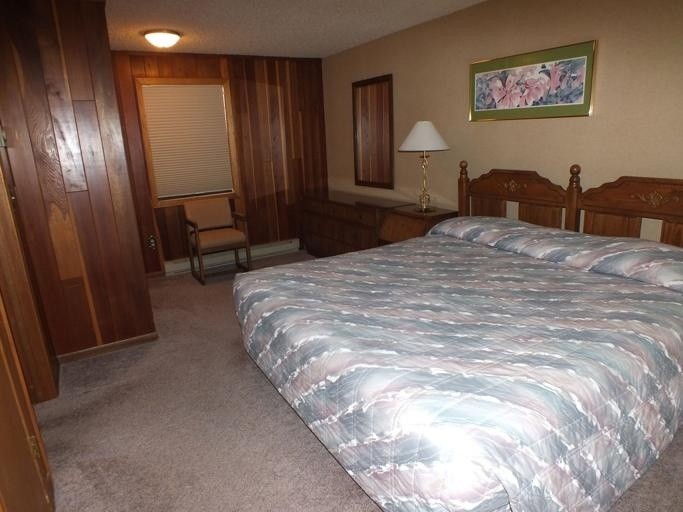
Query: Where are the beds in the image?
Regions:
[232,156,682,512]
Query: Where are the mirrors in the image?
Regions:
[351,74,395,188]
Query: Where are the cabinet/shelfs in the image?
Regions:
[298,191,458,260]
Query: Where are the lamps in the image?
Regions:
[397,119,452,214]
[142,29,182,50]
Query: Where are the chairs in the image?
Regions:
[183,196,253,287]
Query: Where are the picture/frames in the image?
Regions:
[467,38,600,123]
